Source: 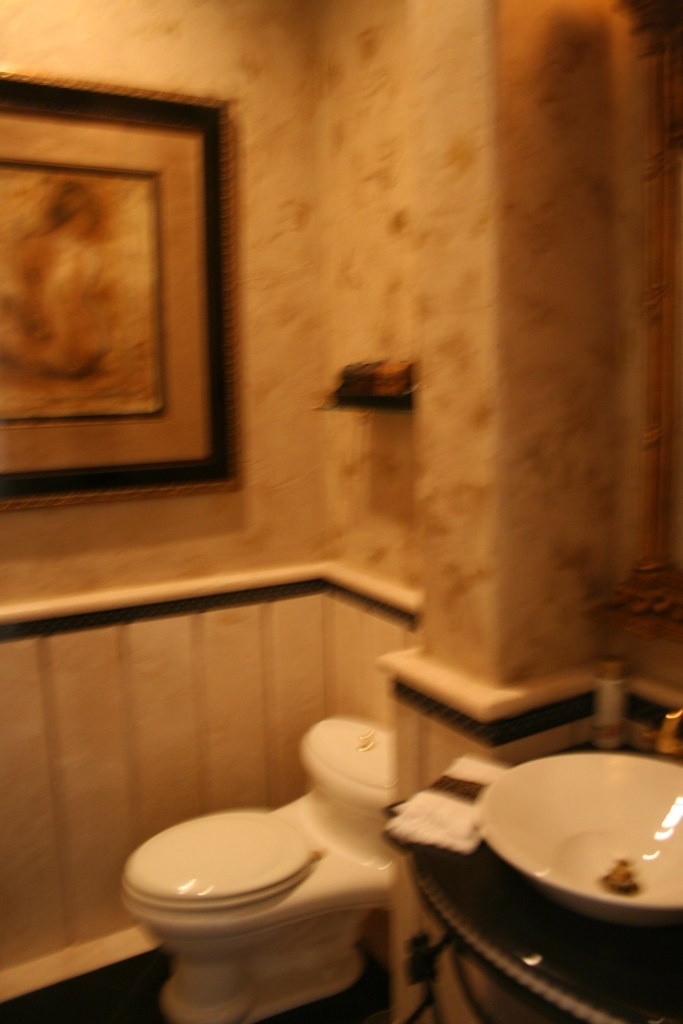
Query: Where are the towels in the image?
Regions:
[383,756,505,856]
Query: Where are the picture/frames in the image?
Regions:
[0,72,247,516]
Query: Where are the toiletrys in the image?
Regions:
[594,661,624,747]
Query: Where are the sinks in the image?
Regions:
[477,752,683,909]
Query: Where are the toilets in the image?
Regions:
[120,717,396,1024]
